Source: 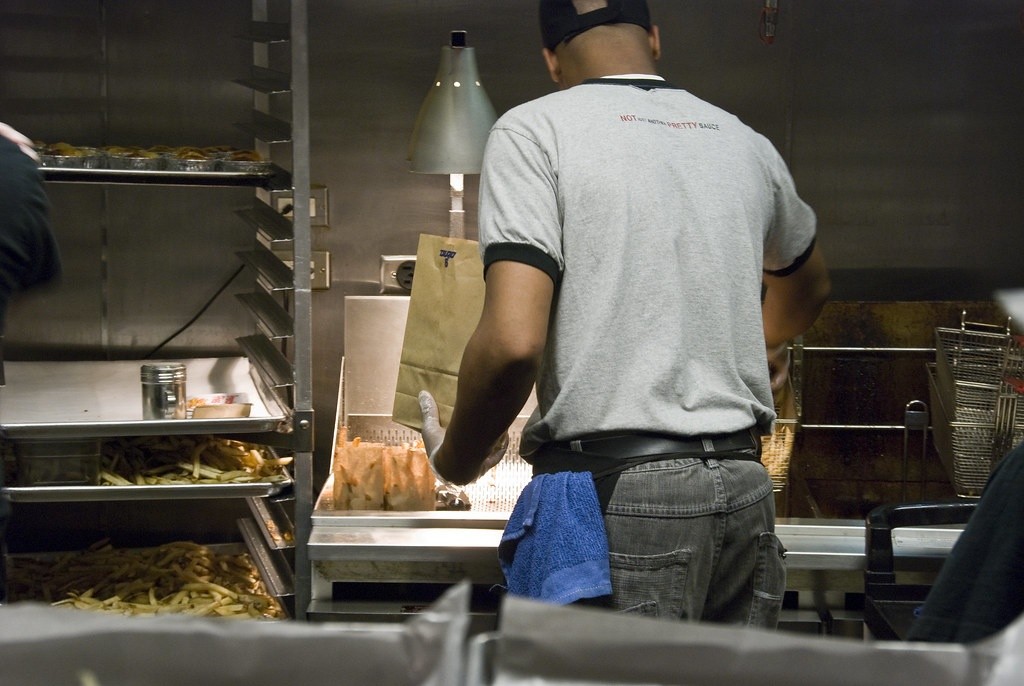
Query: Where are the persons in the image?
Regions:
[419,0,831,630]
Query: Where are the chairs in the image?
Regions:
[854,443,1024,646]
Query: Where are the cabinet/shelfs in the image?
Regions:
[0,0,315,620]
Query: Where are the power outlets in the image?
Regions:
[274,186,330,228]
[274,251,332,290]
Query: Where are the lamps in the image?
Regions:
[406,29,499,237]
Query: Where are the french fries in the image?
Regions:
[8,534,282,621]
[97,435,294,486]
[758,424,794,477]
[334,427,435,511]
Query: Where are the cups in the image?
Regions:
[139,362,187,420]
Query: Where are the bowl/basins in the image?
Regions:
[39,147,271,173]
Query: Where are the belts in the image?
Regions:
[548,429,757,460]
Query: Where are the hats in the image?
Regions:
[539,0,651,51]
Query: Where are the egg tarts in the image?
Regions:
[40,139,273,171]
